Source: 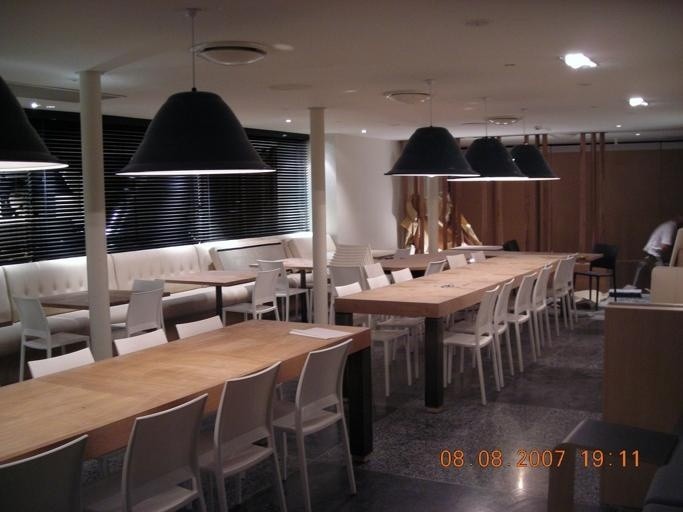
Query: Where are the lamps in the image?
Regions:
[383,79,480,178]
[189,42,268,66]
[114,8,277,179]
[0,77,70,172]
[384,90,433,107]
[509,107,561,180]
[446,95,529,181]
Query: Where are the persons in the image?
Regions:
[633,214,683,288]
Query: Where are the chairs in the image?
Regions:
[573,242,618,311]
[549,413,683,511]
